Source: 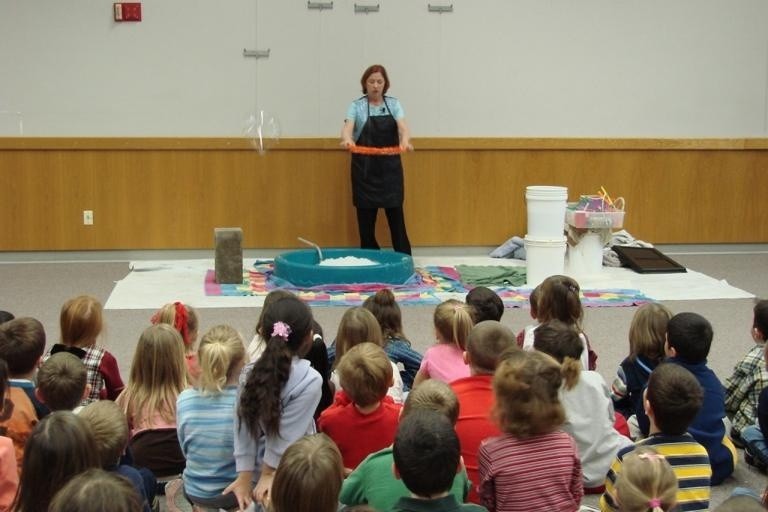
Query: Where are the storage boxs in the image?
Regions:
[565,201,625,229]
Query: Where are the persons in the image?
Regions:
[340,65,414,258]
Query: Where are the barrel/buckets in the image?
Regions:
[524,234,568,289]
[568,232,603,274]
[523,186,569,239]
